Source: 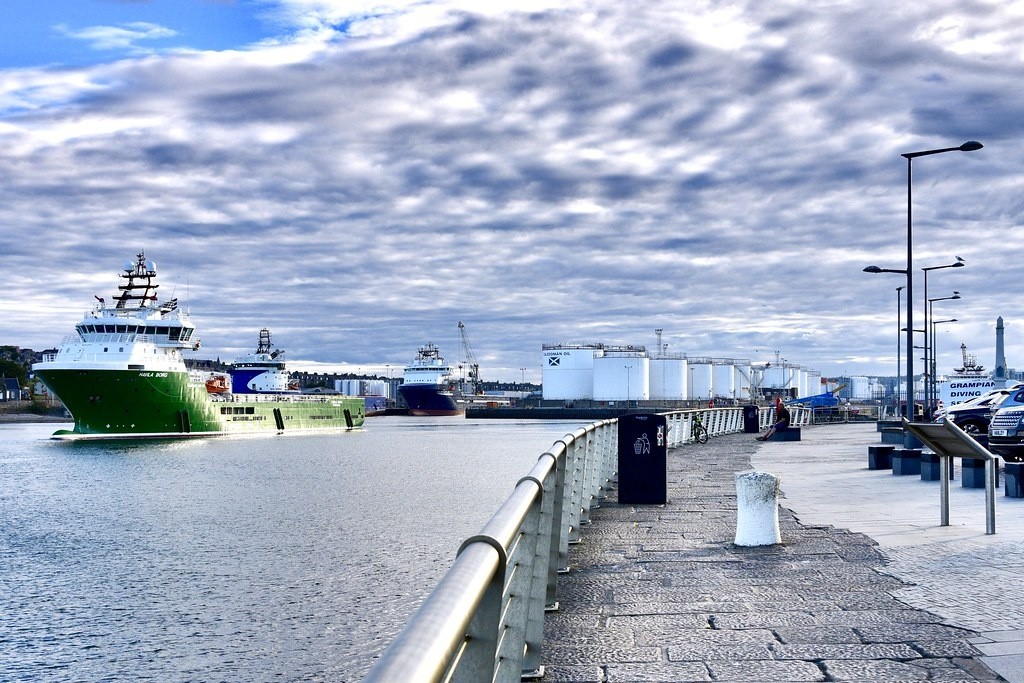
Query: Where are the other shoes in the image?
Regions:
[756,436,768,441]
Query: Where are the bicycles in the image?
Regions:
[692,411,708,444]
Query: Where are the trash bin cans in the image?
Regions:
[744,405,762,433]
[901,403,924,420]
[618,413,667,505]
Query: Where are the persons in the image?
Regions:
[756,403,790,441]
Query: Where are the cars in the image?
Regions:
[931,382,1024,461]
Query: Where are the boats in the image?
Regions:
[29,252,367,445]
[396,343,468,417]
[188,326,396,418]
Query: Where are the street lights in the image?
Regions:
[863,140,984,426]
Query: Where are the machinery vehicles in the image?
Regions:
[456,320,484,396]
